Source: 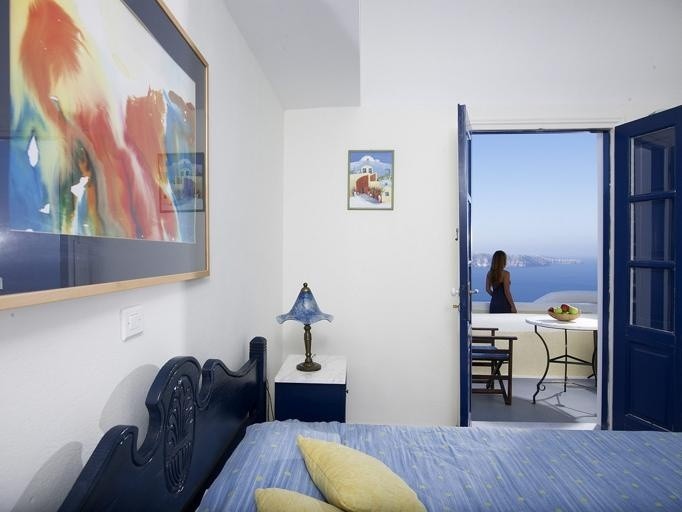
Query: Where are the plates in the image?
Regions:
[547,311,581,321]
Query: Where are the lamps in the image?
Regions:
[275,282,334,371]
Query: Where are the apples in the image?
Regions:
[549,304,578,315]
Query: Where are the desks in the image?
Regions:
[526,318,598,404]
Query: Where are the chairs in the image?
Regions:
[471,328,518,406]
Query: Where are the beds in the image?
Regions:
[57,336,682,512]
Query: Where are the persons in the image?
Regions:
[486,250,517,313]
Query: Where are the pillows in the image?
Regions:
[193,419,427,512]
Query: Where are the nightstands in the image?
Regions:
[274,354,348,423]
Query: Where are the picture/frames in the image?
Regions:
[348,150,394,210]
[0,0,210,311]
